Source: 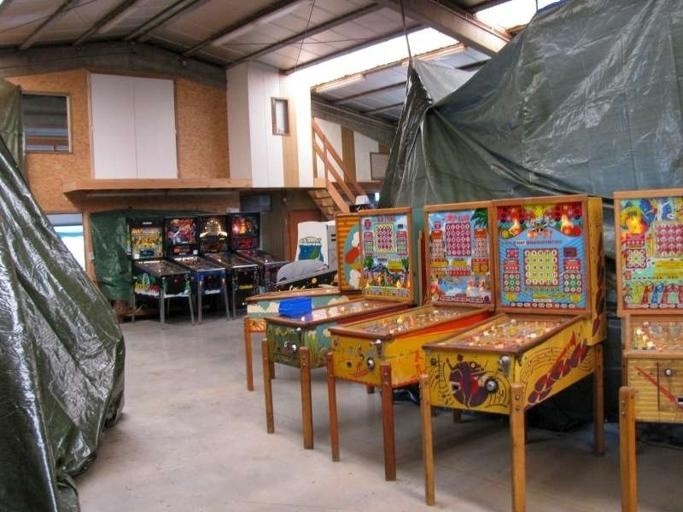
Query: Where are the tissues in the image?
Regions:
[349,195,373,212]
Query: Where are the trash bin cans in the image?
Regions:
[274,259,337,291]
